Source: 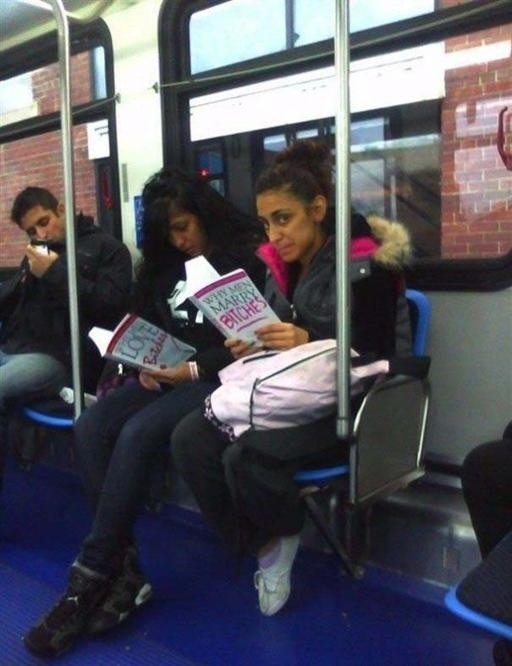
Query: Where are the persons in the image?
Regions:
[167,139,415,620]
[23,164,269,659]
[0,183,133,405]
[458,419,512,665]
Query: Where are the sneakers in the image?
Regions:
[88,561,152,636]
[23,563,109,656]
[254,532,302,616]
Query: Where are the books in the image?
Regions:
[84,307,196,378]
[182,253,281,349]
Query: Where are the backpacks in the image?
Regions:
[201,338,390,442]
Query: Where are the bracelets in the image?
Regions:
[189,359,200,381]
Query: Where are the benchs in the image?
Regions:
[18,289,430,582]
